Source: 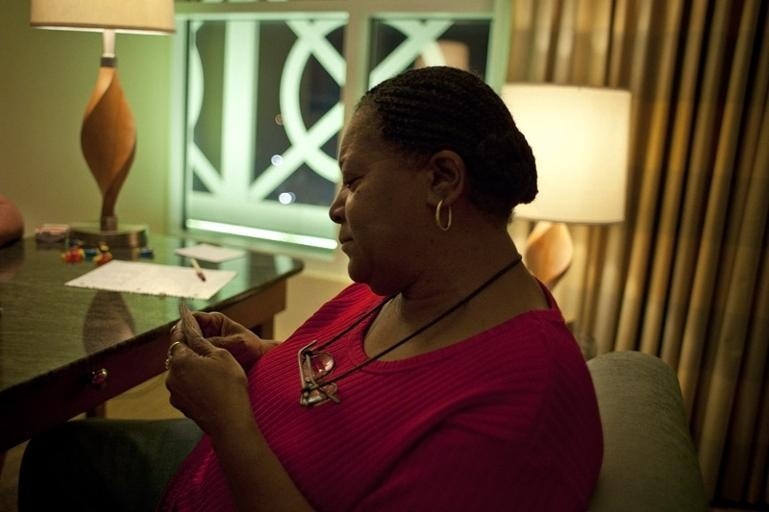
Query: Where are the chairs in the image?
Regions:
[582,351,708,512]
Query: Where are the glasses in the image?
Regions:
[297,338,341,408]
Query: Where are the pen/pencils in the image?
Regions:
[191,257,205,282]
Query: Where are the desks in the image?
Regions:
[0,230,306,512]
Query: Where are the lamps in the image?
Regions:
[499,81,632,293]
[28,1,176,250]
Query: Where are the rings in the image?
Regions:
[166,341,179,369]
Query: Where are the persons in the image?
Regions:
[18,67,603,512]
